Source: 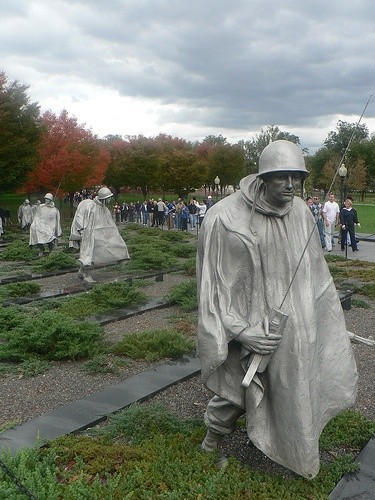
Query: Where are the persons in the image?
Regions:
[68,186,131,288]
[113,195,215,233]
[29,192,62,256]
[0,218,4,237]
[4,207,13,229]
[63,187,97,207]
[195,139,360,481]
[17,199,41,232]
[306,190,361,253]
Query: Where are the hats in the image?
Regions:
[158,198,162,201]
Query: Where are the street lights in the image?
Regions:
[214,175,221,203]
[338,163,348,250]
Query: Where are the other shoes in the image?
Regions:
[327,250,331,252]
[353,249,359,252]
[339,242,341,243]
[341,245,345,251]
[356,241,359,243]
[348,244,351,246]
[322,244,326,248]
[333,244,336,247]
